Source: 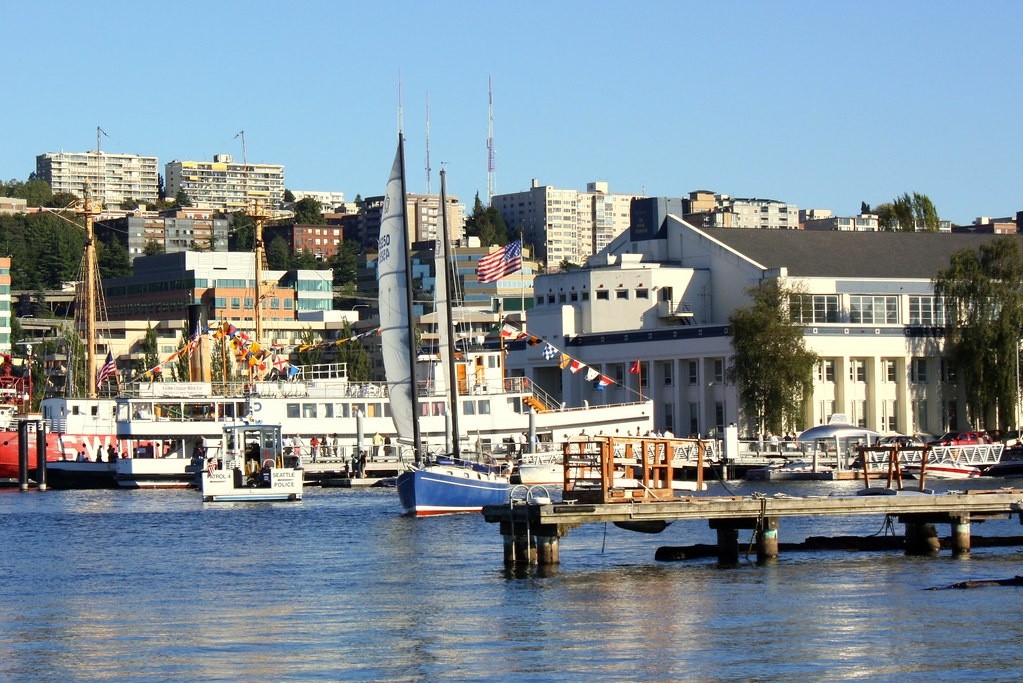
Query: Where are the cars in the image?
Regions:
[876,433,935,447]
[992,431,1023,449]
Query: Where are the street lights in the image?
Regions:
[1016,339,1023,438]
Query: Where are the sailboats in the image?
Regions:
[119,219,349,398]
[379,130,512,520]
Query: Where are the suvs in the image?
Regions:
[927,430,994,448]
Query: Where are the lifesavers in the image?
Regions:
[254,402,262,411]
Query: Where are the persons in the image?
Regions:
[484,430,718,477]
[77,443,215,475]
[769,431,1023,469]
[282,432,392,478]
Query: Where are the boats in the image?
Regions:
[0,349,656,492]
[518,458,641,484]
[905,458,982,480]
[983,460,1023,478]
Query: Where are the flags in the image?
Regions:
[96,350,117,391]
[143,320,381,377]
[489,321,614,391]
[477,239,521,284]
[628,360,640,374]
